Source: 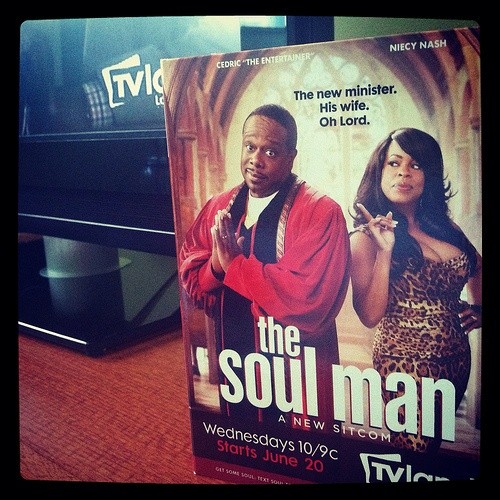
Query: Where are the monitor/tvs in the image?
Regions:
[17,15,334,261]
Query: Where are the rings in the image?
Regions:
[470,315,478,321]
[221,235,229,240]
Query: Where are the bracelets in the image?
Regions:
[468,304,483,314]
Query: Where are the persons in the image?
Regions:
[178,103,351,434]
[349,127,483,454]
